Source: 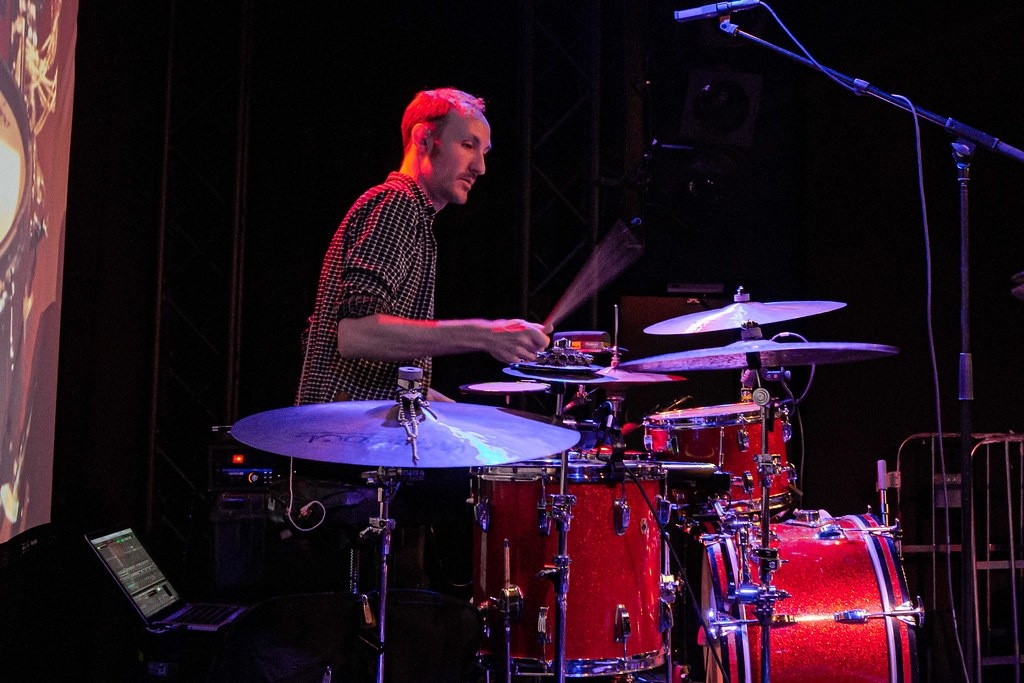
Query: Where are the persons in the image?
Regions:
[295,87,553,643]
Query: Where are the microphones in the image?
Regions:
[592,402,609,422]
[674,0,760,23]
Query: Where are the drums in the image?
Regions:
[468,462,669,677]
[460,377,558,417]
[660,510,924,683]
[0,54,44,526]
[642,396,793,517]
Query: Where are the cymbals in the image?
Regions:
[642,299,848,336]
[616,341,900,370]
[511,360,602,373]
[502,365,688,384]
[230,401,583,470]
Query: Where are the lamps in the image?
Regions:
[699,0,765,50]
[678,67,764,149]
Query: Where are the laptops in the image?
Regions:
[86,527,247,631]
